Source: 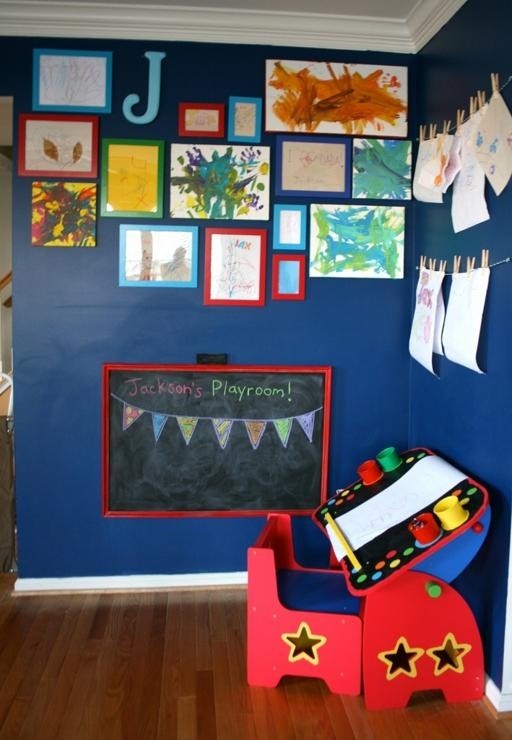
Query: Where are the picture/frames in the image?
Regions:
[176,101,226,138]
[271,253,307,301]
[30,47,113,115]
[274,133,352,199]
[272,204,307,251]
[202,225,269,307]
[15,111,99,180]
[115,220,198,290]
[226,95,264,145]
[98,138,165,219]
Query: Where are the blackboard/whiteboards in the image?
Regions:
[100,364,332,519]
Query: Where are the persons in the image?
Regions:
[159,246,191,282]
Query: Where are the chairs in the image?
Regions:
[240,509,365,703]
